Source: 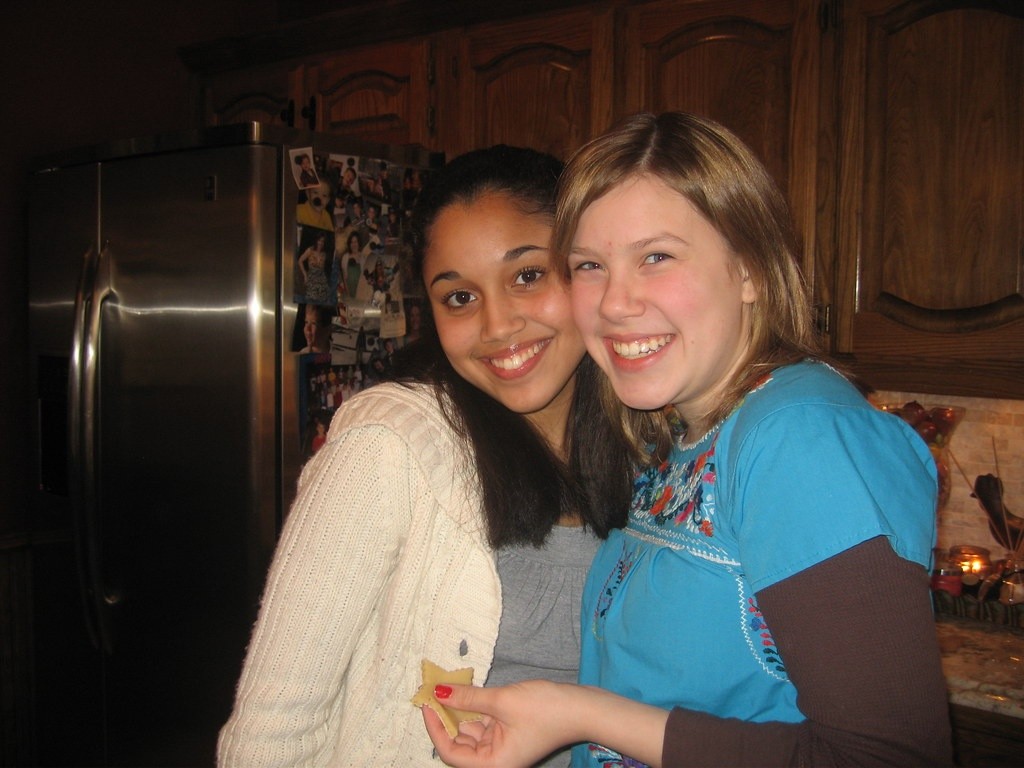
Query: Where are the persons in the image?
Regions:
[420,108,956,768]
[213,145,631,768]
[292,150,420,458]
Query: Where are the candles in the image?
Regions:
[932,544,994,597]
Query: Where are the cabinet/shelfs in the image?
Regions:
[176,0,1024,400]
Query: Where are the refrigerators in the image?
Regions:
[12,119,443,768]
